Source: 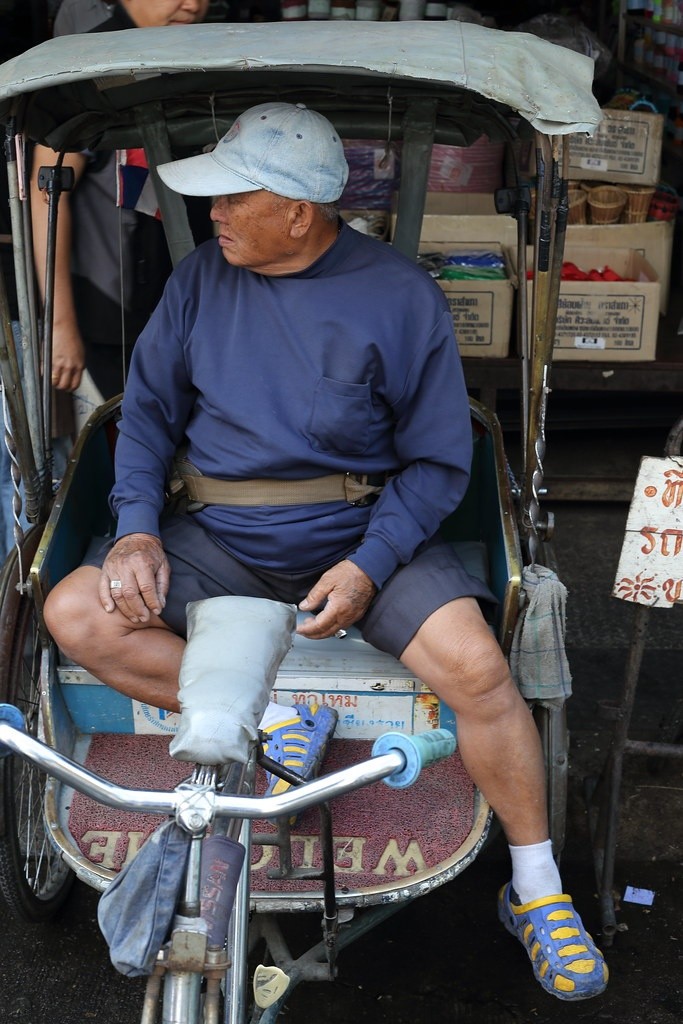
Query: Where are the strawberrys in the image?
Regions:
[525,262,628,282]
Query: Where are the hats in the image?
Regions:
[156,102,349,203]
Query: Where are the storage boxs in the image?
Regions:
[393,113,673,362]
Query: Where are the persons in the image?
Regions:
[565,8,619,107]
[30,0,214,401]
[43,102,609,1001]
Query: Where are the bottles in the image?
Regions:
[626,0,683,146]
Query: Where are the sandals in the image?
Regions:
[256,703,339,829]
[499,881,610,1002]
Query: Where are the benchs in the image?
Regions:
[25,385,527,745]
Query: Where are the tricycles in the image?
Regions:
[0,19,606,1024]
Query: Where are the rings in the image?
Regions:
[110,581,122,589]
[334,629,348,639]
